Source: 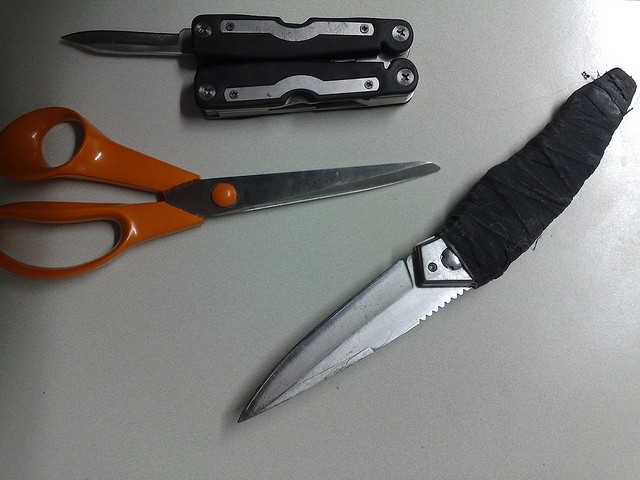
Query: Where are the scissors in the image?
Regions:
[0,106,441,280]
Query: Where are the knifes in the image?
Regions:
[236,65,638,425]
[60,12,420,122]
